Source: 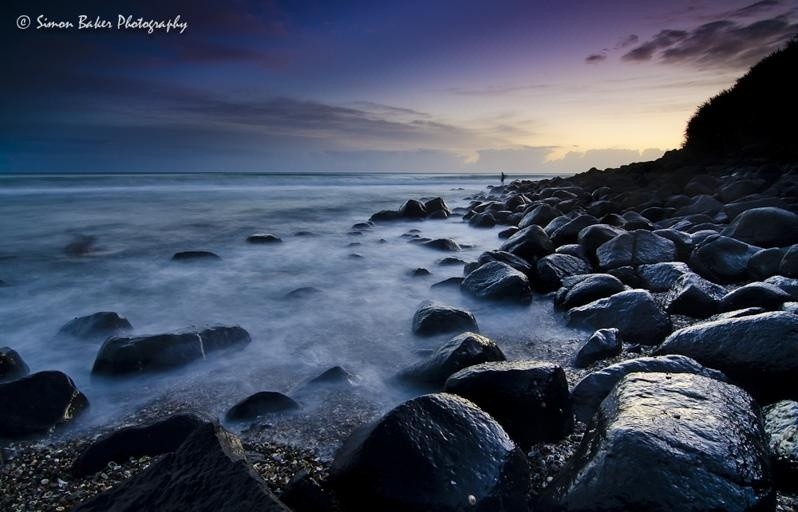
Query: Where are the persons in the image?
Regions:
[500,171,505,185]
